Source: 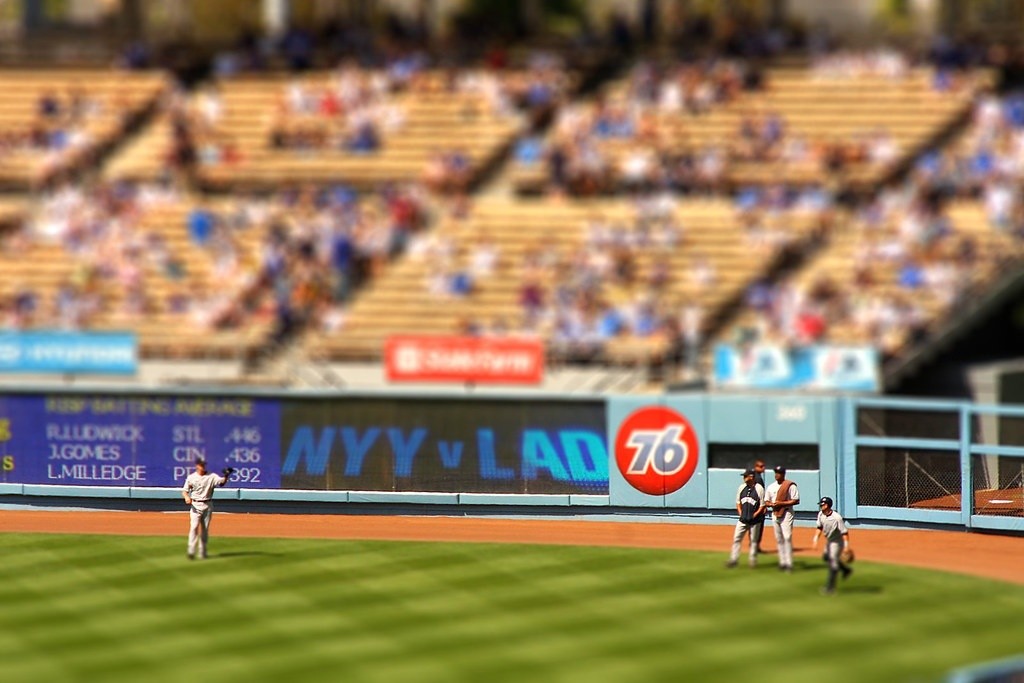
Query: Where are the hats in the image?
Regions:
[194,458,208,464]
[741,470,755,477]
[774,465,786,474]
[818,495,832,508]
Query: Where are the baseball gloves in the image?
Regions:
[840,549,854,562]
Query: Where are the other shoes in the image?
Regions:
[780,564,793,574]
[821,587,835,594]
[728,560,736,567]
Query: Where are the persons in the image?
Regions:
[182,457,233,560]
[813,497,853,591]
[726,460,801,571]
